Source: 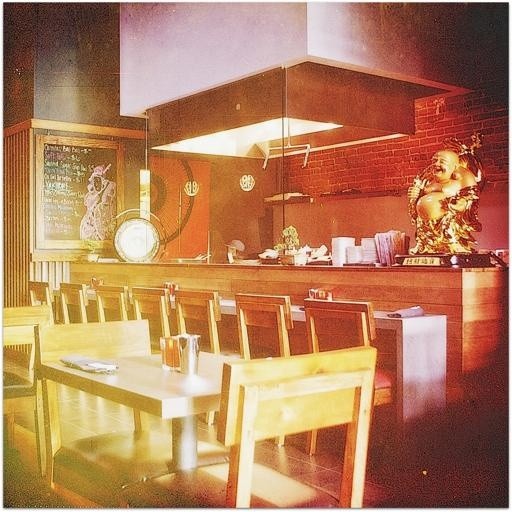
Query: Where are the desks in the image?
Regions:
[53,286,448,426]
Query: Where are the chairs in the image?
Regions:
[234,294,308,446]
[174,290,223,425]
[28,280,52,306]
[33,318,231,508]
[3,305,54,477]
[59,283,89,324]
[301,297,396,456]
[115,345,378,509]
[131,286,171,354]
[95,284,130,323]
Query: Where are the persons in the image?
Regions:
[407,150,478,254]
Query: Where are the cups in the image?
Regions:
[160,335,180,370]
[308,288,332,300]
[177,332,201,374]
[163,282,179,295]
[293,254,307,266]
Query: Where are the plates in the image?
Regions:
[59,354,118,373]
[330,236,376,267]
